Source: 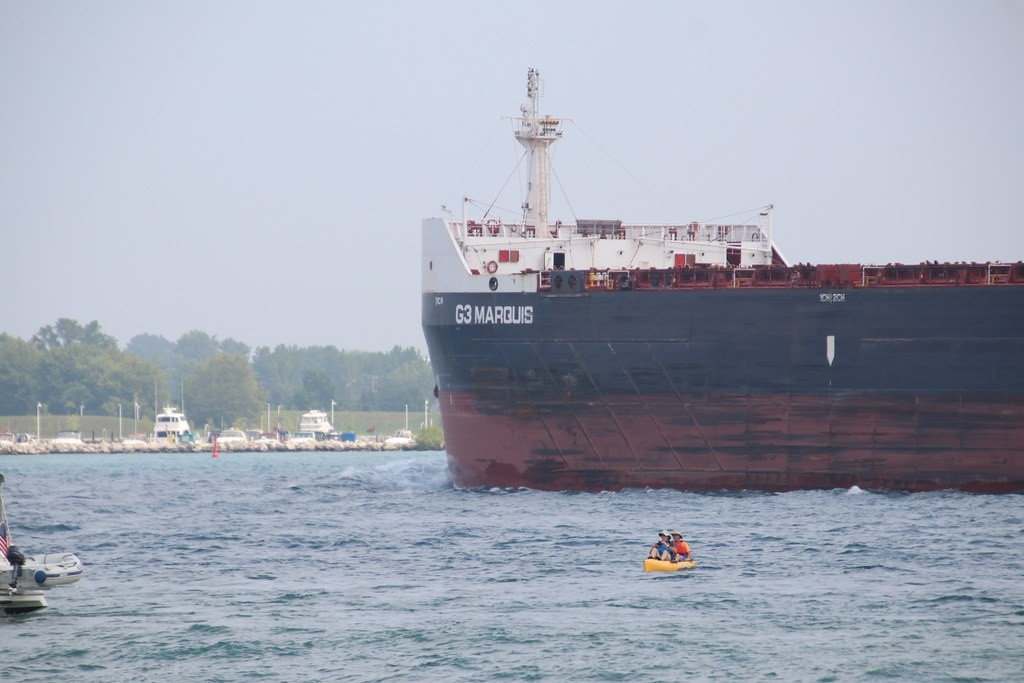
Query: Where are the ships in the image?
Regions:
[420,67,1024,493]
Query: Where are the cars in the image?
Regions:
[207,430,284,443]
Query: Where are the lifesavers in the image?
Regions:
[617,274,632,290]
[487,260,498,273]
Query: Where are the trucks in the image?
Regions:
[155,405,191,442]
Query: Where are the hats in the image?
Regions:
[671,530,683,540]
[658,530,671,539]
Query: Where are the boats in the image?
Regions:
[644,559,695,572]
[0,474,83,614]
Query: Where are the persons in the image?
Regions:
[647,530,691,562]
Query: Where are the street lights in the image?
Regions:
[135,402,141,433]
[332,400,337,427]
[424,399,428,429]
[266,403,270,433]
[117,404,122,439]
[37,401,42,440]
[404,405,408,430]
[81,405,84,417]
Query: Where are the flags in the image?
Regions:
[0,520,10,557]
